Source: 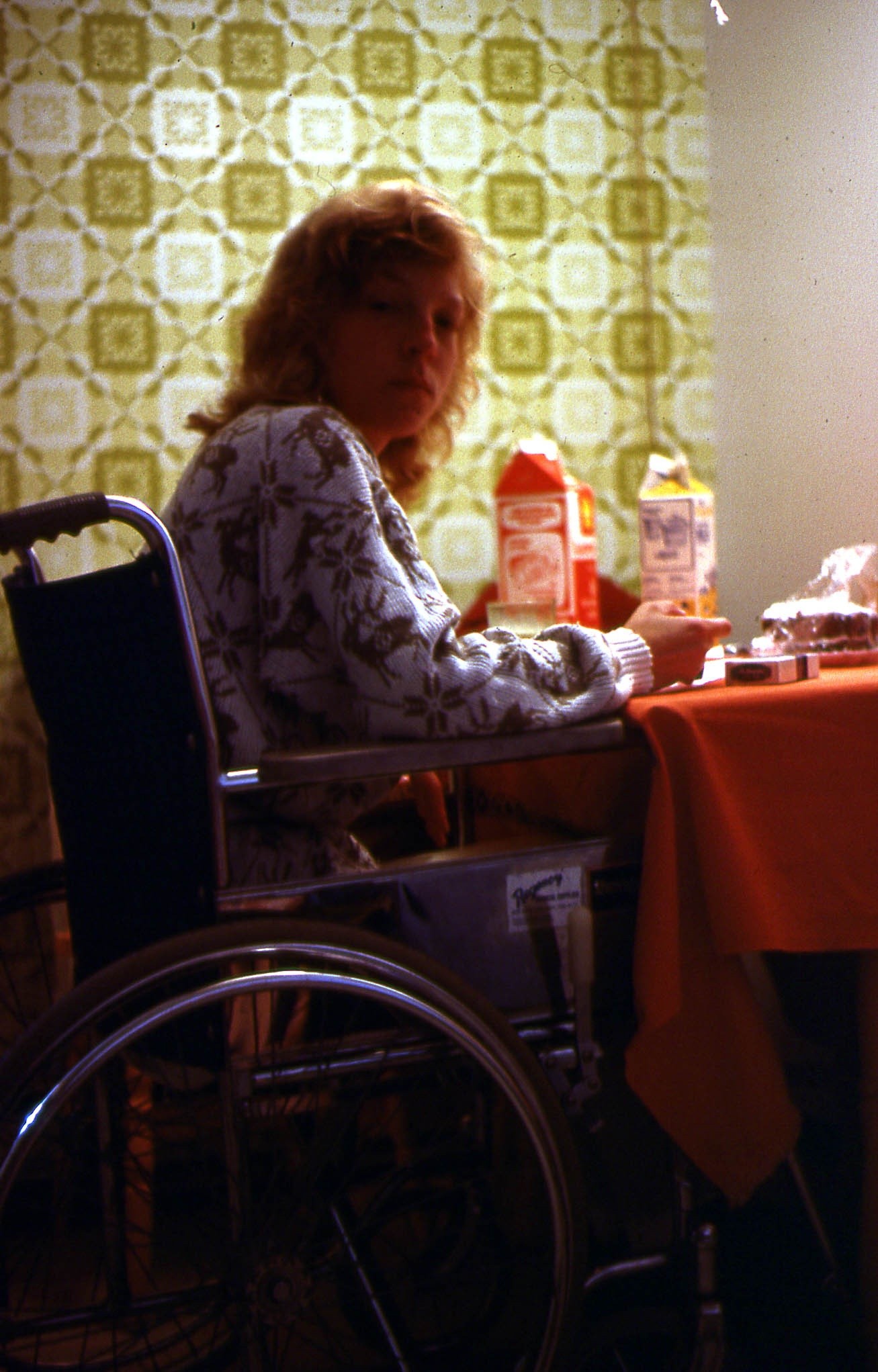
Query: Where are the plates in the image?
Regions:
[758,643,877,668]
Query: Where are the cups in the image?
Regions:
[483,595,557,638]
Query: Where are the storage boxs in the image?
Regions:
[726,654,818,687]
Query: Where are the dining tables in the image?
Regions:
[466,666,878,1304]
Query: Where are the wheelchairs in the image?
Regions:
[0,489,728,1371]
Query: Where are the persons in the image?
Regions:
[157,180,727,865]
